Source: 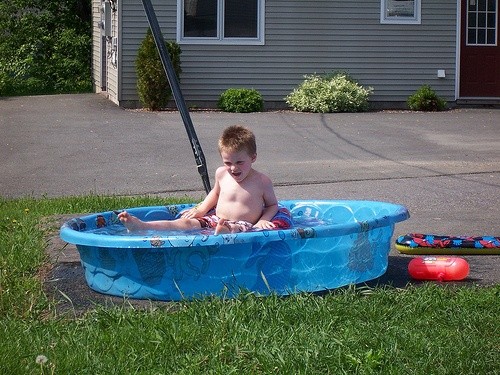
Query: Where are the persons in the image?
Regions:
[118,125,279,232]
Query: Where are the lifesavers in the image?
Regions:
[395,233,500,255]
[180,208,292,231]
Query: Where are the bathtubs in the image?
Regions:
[60,199,410,301]
